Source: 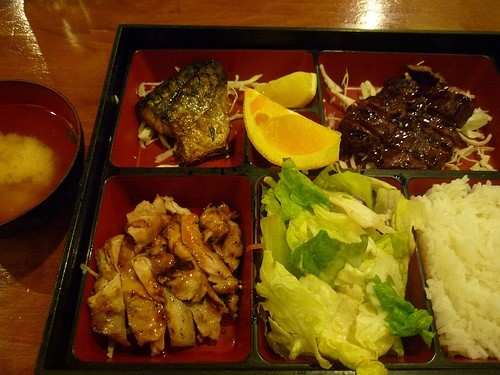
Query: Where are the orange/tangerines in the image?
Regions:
[243,70,343,169]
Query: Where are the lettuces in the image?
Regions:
[256,157,436,375]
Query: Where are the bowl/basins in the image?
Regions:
[0,80,84,240]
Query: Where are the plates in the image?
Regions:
[32,23,497,374]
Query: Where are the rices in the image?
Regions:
[410,174,499,362]
